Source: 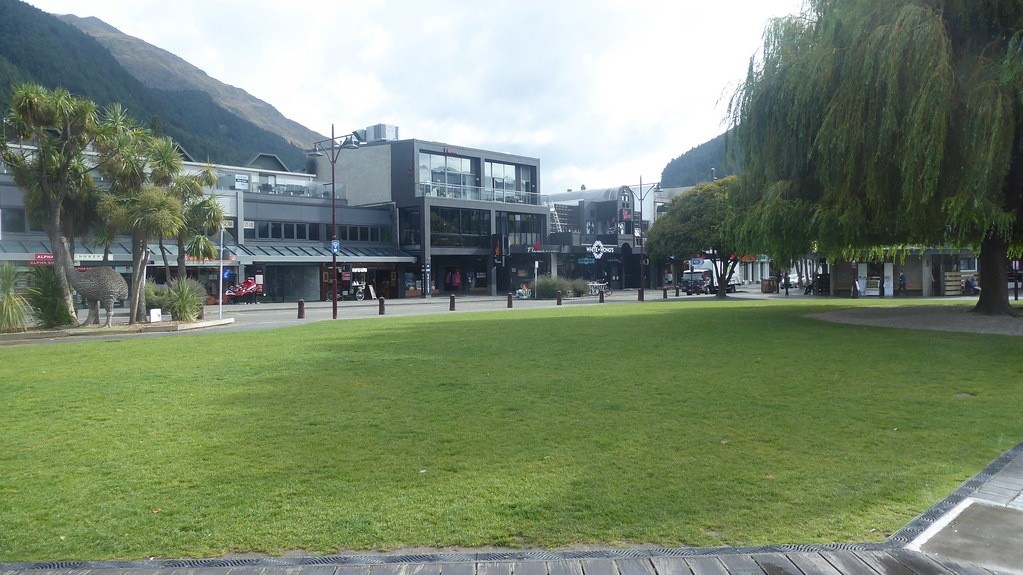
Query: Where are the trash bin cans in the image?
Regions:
[761,279,774,293]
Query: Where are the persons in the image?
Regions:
[784,273,791,295]
[667,271,673,291]
[446,271,461,291]
[896,271,908,296]
[965,275,981,295]
[804,280,816,296]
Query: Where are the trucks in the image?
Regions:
[679,257,741,295]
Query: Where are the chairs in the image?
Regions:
[257,184,310,196]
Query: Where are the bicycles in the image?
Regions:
[586,278,614,296]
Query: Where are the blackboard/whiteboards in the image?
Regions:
[368,285,376,298]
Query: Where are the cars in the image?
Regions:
[779,273,806,289]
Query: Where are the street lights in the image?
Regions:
[1012,255,1020,301]
[306,123,358,319]
[620,175,664,301]
